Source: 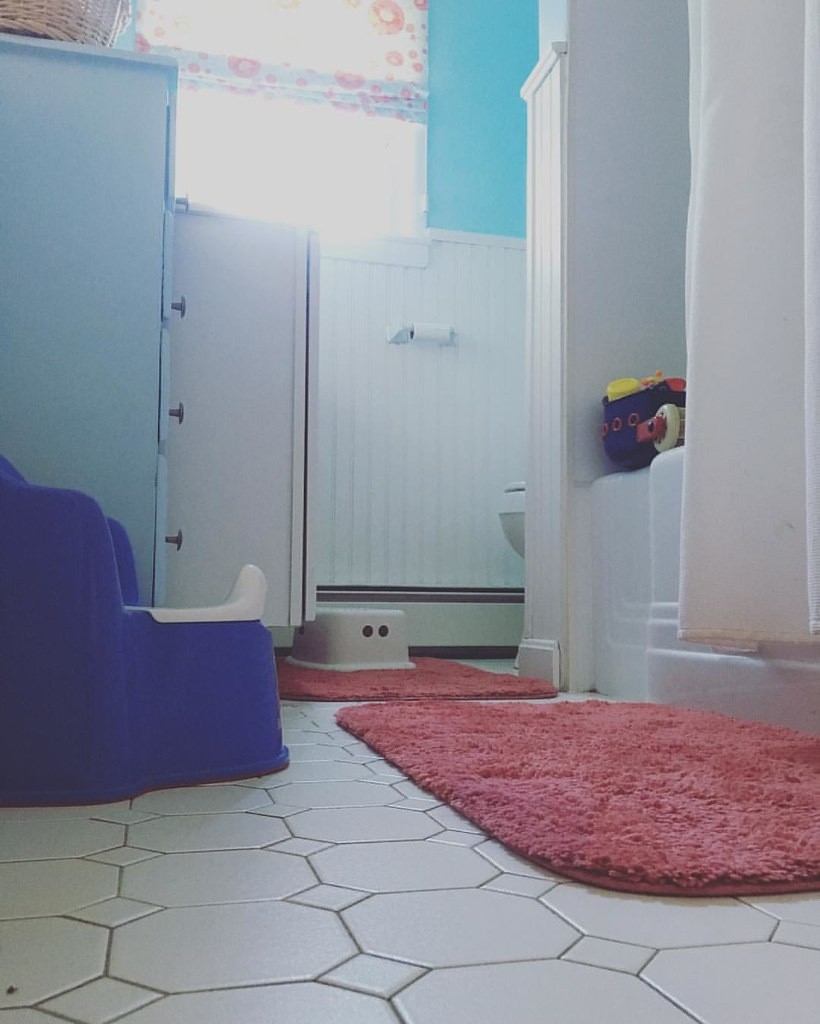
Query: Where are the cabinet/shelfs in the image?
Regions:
[0,33,189,607]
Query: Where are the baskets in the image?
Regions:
[0,0,131,49]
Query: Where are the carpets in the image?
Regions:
[274,655,558,700]
[336,701,820,899]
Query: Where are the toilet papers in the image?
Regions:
[407,321,451,342]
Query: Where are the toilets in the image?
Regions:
[498,482,525,556]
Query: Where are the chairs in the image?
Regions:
[0,455,289,806]
